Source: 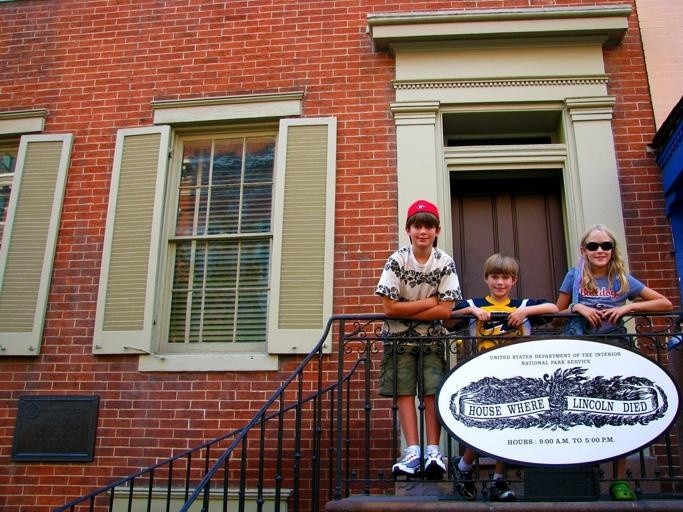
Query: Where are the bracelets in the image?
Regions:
[566,302,578,315]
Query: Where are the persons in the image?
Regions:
[373,199,463,482]
[551,224,674,501]
[442,253,559,501]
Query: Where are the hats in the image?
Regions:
[406,201,439,247]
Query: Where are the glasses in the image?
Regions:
[585,243,612,250]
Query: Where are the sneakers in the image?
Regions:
[391,449,515,502]
[610,480,636,500]
